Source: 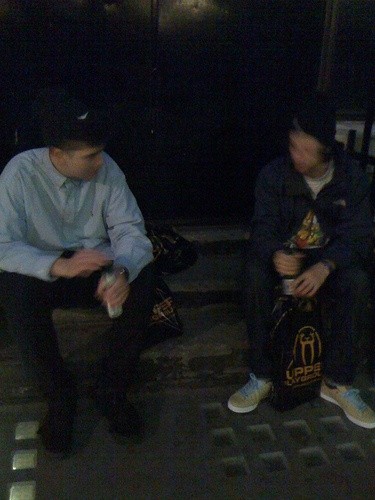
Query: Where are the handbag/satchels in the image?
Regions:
[148,227,198,273]
[148,275,184,342]
[262,295,327,412]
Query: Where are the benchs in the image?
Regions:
[52,280,240,305]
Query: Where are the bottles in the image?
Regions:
[101,260,123,319]
[280,240,302,299]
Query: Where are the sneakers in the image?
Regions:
[226,376,274,415]
[319,377,375,430]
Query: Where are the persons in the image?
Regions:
[227,97,375,430]
[0,100,159,452]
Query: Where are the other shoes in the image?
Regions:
[38,396,80,460]
[95,385,147,444]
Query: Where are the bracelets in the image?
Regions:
[320,259,333,273]
